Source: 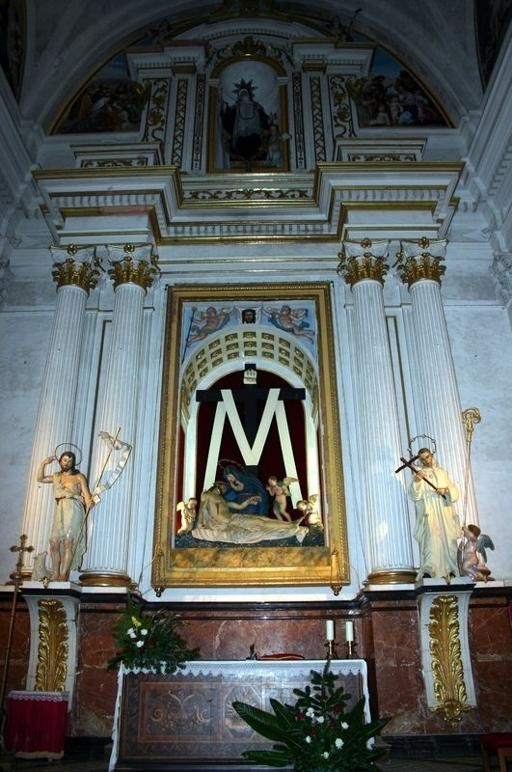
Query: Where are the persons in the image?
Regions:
[369,75,428,125]
[407,446,463,579]
[263,123,280,161]
[186,306,226,348]
[36,451,96,581]
[177,474,323,544]
[270,305,315,336]
[460,524,489,583]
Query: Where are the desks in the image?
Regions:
[5,687,71,761]
[105,656,387,770]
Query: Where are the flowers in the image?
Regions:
[101,589,196,676]
[231,656,398,772]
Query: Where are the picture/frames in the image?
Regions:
[145,278,354,596]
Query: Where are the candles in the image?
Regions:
[325,618,335,640]
[344,620,354,641]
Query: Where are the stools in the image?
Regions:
[476,732,512,771]
